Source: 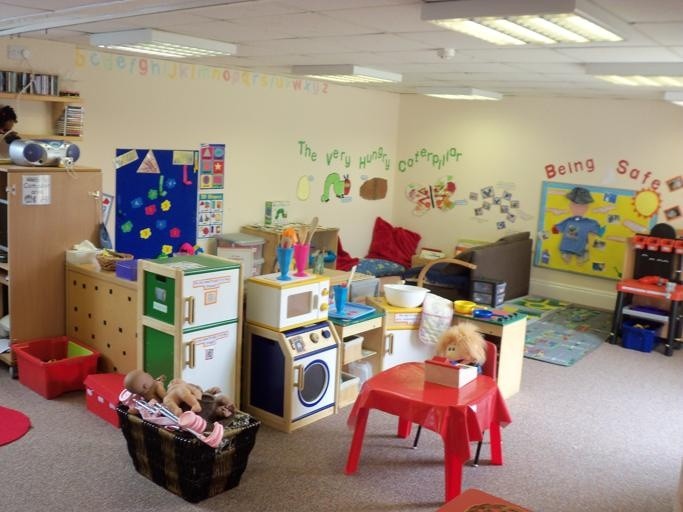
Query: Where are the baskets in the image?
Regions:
[95,253,133,271]
[115,402,261,503]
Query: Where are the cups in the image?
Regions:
[293,244,310,277]
[332,285,349,316]
[275,247,292,280]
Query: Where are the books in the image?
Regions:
[53,102,86,138]
[0,70,79,99]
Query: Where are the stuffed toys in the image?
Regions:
[437,321,485,378]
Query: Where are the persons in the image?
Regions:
[122,368,168,405]
[204,386,237,418]
[162,376,203,417]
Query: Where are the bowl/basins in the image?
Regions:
[382,284,431,309]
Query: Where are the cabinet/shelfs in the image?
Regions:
[363,295,423,371]
[328,310,384,414]
[0,163,105,379]
[471,276,506,308]
[135,252,244,410]
[64,250,138,381]
[0,91,83,141]
[239,222,338,274]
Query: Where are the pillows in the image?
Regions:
[363,216,421,269]
[335,236,358,271]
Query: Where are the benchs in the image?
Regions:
[401,232,533,305]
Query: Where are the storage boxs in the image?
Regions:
[10,334,99,400]
[620,318,663,352]
[81,373,126,429]
[215,231,265,262]
[251,258,264,277]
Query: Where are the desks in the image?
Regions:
[452,308,528,401]
[434,486,531,512]
[344,362,511,506]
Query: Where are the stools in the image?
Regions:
[352,258,404,278]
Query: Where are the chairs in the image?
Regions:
[411,342,499,468]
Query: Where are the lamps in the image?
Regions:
[415,86,503,101]
[583,61,682,92]
[419,1,631,51]
[290,63,403,85]
[88,28,237,62]
[662,91,682,107]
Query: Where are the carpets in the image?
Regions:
[0,405,33,448]
[494,293,614,368]
